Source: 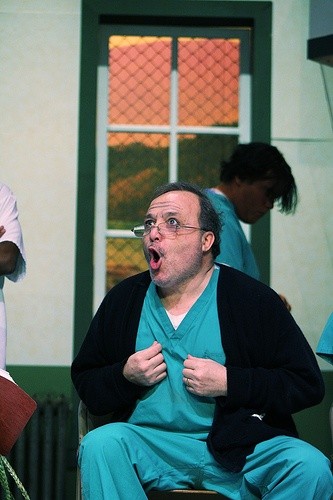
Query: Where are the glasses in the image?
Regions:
[129,221,207,238]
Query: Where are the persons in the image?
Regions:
[67,181,333,500]
[0,178,29,372]
[193,140,300,314]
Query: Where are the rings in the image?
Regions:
[184,378,191,387]
